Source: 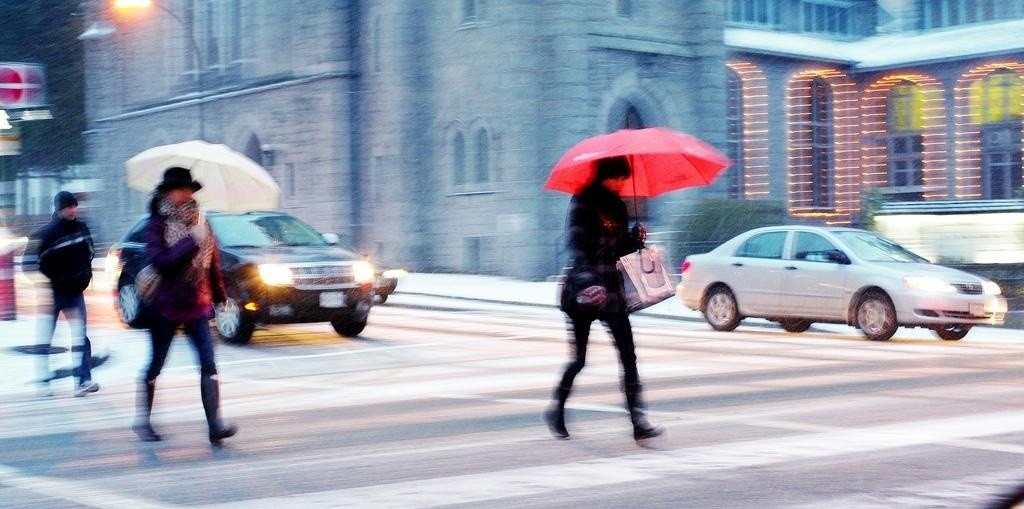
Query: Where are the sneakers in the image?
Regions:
[74,381,98,397]
[545,406,569,440]
[634,423,665,440]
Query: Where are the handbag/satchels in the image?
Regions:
[615,244,675,313]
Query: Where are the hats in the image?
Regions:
[54,190,78,211]
[157,168,201,193]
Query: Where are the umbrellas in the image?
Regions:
[123,139,280,221]
[545,126,733,274]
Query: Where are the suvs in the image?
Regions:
[111,212,370,344]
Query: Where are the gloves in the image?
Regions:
[577,286,608,306]
[631,222,648,244]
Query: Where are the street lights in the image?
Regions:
[114,0,208,141]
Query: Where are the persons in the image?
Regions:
[544,156,665,440]
[23,191,99,396]
[118,166,238,445]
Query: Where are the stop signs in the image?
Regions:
[0,63,48,109]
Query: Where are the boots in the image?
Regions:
[131,382,162,442]
[204,381,238,445]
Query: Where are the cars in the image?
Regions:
[675,224,1008,342]
[320,231,401,311]
